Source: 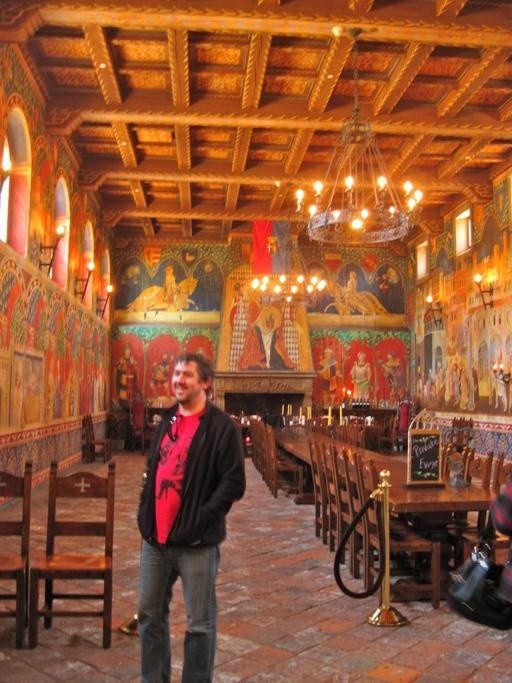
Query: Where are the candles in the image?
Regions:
[282,402,343,427]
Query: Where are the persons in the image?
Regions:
[490,481,512,603]
[348,271,357,294]
[252,287,296,371]
[166,265,178,299]
[415,360,511,413]
[113,343,211,403]
[137,354,244,683]
[318,348,404,405]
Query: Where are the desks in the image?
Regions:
[275,425,494,605]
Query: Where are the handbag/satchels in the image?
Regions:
[446,545,512,630]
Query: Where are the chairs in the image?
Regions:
[29,459,116,650]
[0,457,32,645]
[83,413,112,463]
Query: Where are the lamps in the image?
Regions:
[251,218,328,300]
[423,293,443,325]
[493,364,511,384]
[40,226,66,277]
[76,262,95,301]
[97,286,113,317]
[472,274,492,309]
[293,66,422,244]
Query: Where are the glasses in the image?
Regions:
[167,415,183,442]
